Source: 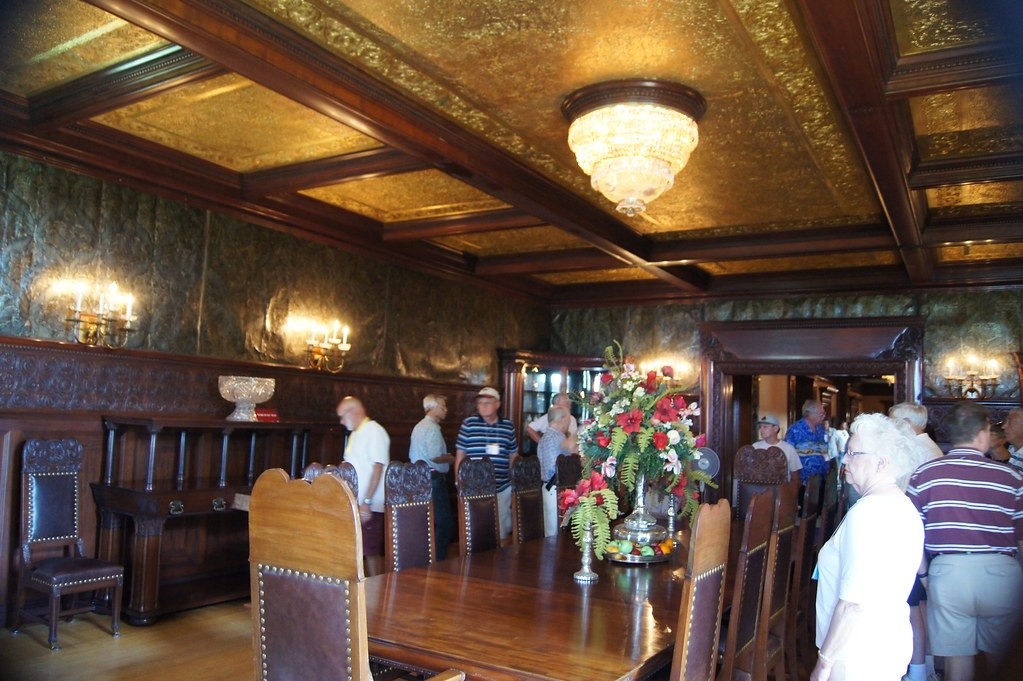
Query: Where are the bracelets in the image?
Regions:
[363,498,372,504]
[918,571,928,578]
[818,650,835,664]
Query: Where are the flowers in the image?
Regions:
[567,364,712,551]
[645,459,702,497]
[552,474,619,549]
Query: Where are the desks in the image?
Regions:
[364,509,798,681]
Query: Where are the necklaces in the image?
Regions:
[863,483,897,496]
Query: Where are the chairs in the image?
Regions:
[556,452,581,535]
[11,436,126,649]
[299,460,363,580]
[654,497,735,681]
[837,461,853,526]
[717,487,776,680]
[718,480,803,680]
[783,475,823,681]
[459,457,500,558]
[812,467,842,581]
[249,464,466,681]
[732,446,788,518]
[512,455,546,545]
[385,459,436,572]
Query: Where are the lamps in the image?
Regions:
[56,268,138,351]
[307,317,353,375]
[564,79,709,218]
[935,344,1001,408]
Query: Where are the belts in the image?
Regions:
[933,551,1016,558]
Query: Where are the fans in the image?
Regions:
[691,447,721,503]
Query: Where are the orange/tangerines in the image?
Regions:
[658,538,673,554]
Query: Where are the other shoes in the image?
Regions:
[926,673,941,681]
[902,676,912,681]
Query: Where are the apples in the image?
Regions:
[609,539,662,556]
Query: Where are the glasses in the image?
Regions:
[847,449,872,458]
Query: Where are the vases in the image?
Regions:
[571,522,602,586]
[607,465,672,565]
[665,492,676,535]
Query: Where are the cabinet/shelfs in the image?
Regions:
[83,413,462,626]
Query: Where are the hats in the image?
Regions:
[475,386,500,401]
[757,415,780,425]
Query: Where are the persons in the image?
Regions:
[810,413,926,681]
[455,387,519,547]
[409,392,455,560]
[752,400,944,526]
[905,402,1023,681]
[336,396,391,575]
[527,391,604,536]
